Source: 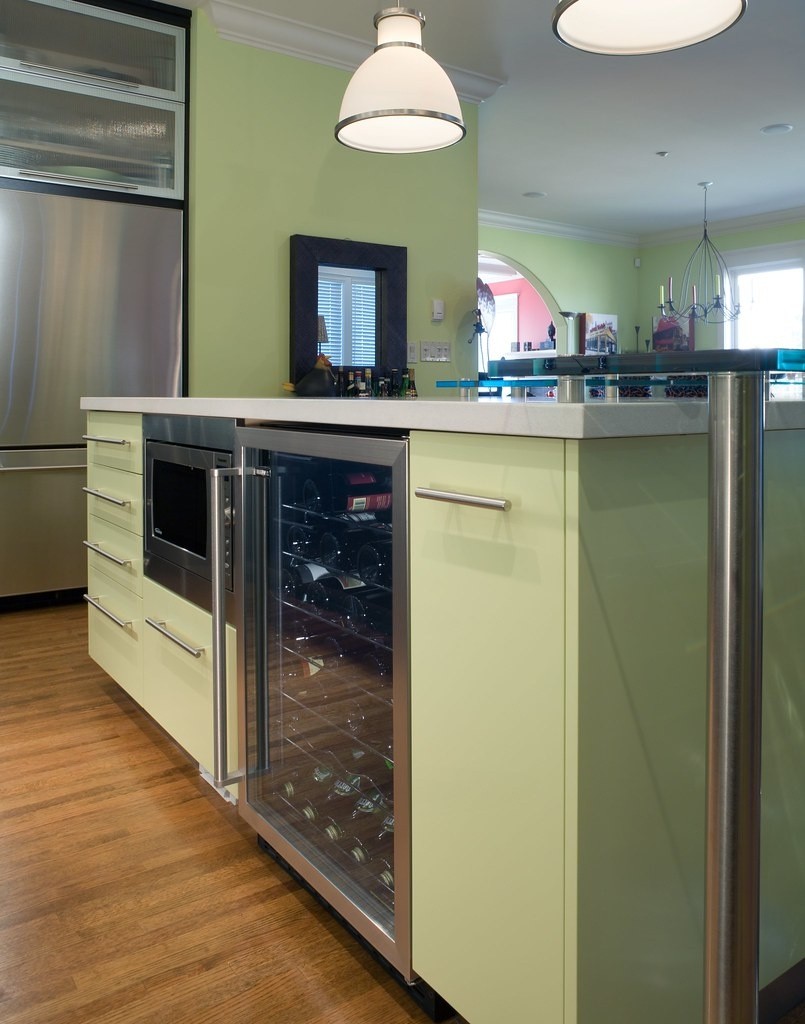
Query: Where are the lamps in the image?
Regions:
[559,312,582,356]
[334,0,467,154]
[550,0,747,58]
[657,182,744,326]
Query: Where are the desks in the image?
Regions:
[433,347,804,1024]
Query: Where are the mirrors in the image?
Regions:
[289,233,408,396]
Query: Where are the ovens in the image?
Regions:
[142,413,245,630]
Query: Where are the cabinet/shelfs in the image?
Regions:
[83,410,238,808]
[403,431,805,1024]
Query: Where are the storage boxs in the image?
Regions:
[579,313,618,355]
[651,314,695,352]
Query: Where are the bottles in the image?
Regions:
[276,480,393,895]
[338,365,418,398]
